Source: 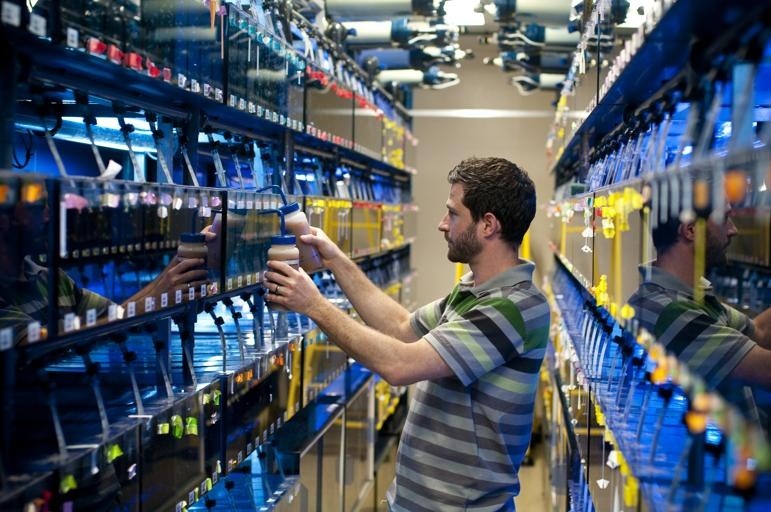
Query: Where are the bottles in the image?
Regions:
[177,232,209,276]
[277,202,325,274]
[268,235,299,312]
[206,201,250,271]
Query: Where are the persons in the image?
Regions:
[263,156,551,511]
[622,199,771,433]
[0,181,246,375]
